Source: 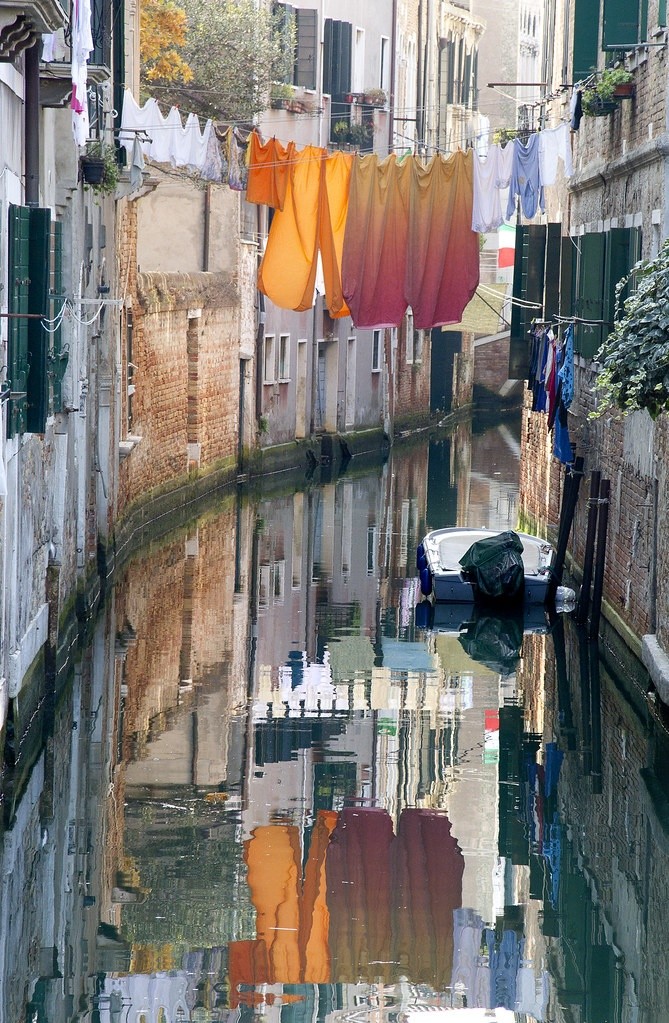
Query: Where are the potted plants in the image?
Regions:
[597,69,635,99]
[581,89,618,117]
[80,140,118,205]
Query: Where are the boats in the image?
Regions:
[411,523,559,607]
[413,592,549,641]
